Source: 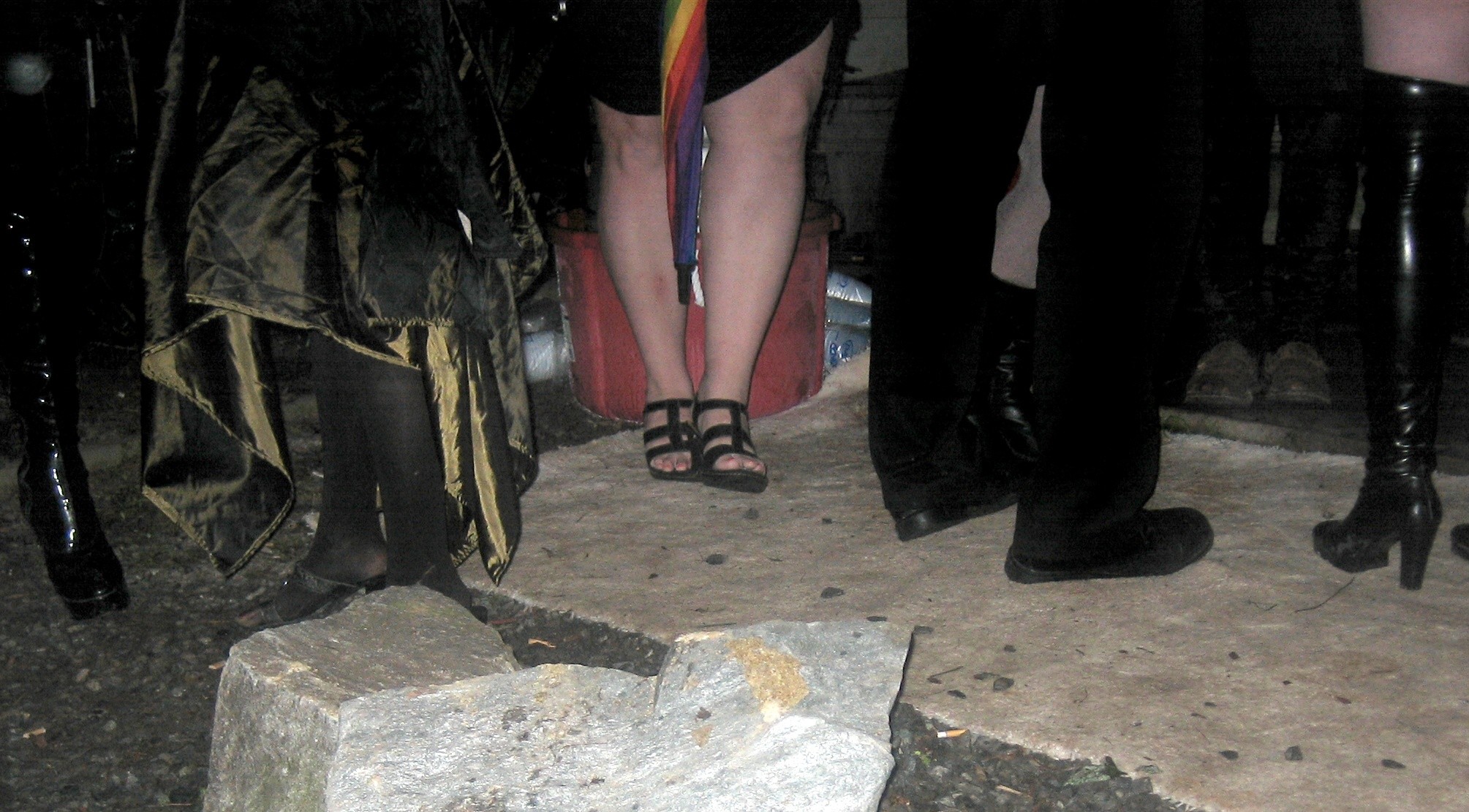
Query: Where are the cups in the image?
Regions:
[824,268,872,372]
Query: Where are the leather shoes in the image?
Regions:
[891,473,1018,540]
[1004,508,1214,585]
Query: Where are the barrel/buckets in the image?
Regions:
[539,193,829,429]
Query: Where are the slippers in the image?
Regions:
[258,560,388,629]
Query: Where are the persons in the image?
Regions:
[0,0,549,630]
[590,0,834,493]
[867,0,1469,590]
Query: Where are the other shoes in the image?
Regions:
[1267,341,1332,408]
[1184,338,1262,410]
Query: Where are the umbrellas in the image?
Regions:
[661,1,708,307]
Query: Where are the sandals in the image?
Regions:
[693,391,769,492]
[642,399,703,482]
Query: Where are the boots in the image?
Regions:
[0,206,129,620]
[1310,60,1469,589]
